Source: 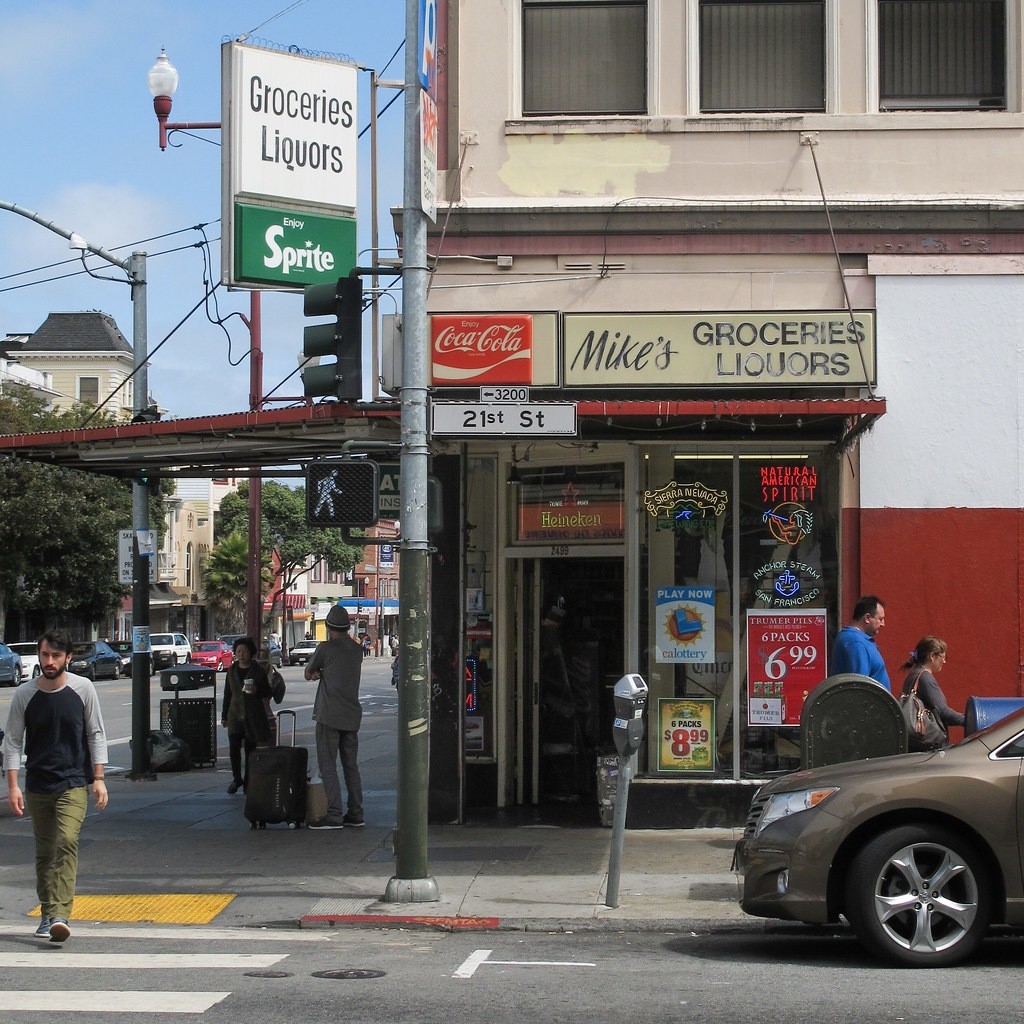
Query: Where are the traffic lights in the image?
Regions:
[301,276,362,402]
[358,603,362,612]
[304,461,380,528]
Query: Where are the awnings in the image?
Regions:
[0,385,889,476]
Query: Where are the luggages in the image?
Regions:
[245,710,308,830]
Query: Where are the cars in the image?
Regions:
[6,642,44,683]
[217,635,283,668]
[289,640,322,665]
[0,642,22,686]
[65,642,122,681]
[190,640,235,671]
[731,707,1024,969]
[105,641,155,678]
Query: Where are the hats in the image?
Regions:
[325,605,351,630]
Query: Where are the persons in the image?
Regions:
[221,638,285,795]
[304,605,365,828]
[390,634,399,656]
[356,633,371,657]
[305,632,313,639]
[902,639,965,752]
[2,630,110,943]
[830,595,891,692]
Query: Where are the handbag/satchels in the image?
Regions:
[899,670,948,751]
[369,645,374,650]
[308,782,327,821]
[271,666,286,704]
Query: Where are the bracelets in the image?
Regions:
[94,776,104,780]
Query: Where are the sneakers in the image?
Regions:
[308,816,343,829]
[343,814,365,826]
[35,919,50,937]
[50,918,71,943]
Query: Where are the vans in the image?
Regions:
[149,633,193,665]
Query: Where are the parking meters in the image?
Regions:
[606,673,649,911]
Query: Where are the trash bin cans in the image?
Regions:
[160,664,217,767]
[596,745,619,826]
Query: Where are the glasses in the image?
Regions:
[934,653,948,660]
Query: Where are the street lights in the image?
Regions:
[364,520,400,657]
[146,46,262,658]
[365,576,369,600]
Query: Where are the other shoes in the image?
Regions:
[227,777,244,794]
